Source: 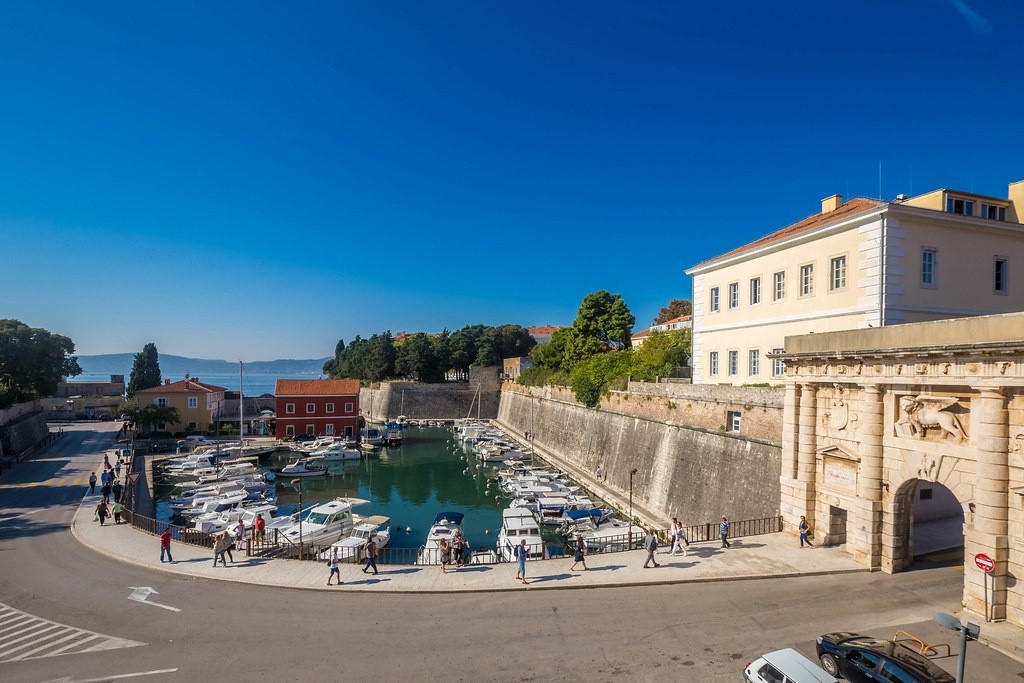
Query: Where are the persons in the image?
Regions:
[101,469,123,504]
[798,515,814,548]
[111,499,123,525]
[212,531,234,568]
[515,538,532,584]
[652,530,659,554]
[439,538,448,572]
[94,499,110,526]
[327,546,344,586]
[451,530,465,568]
[361,537,379,575]
[232,519,245,551]
[642,531,660,569]
[254,514,265,546]
[104,454,121,478]
[89,472,97,494]
[719,515,730,548]
[667,518,689,556]
[569,537,588,571]
[159,527,176,563]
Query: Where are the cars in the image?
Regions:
[293,433,316,441]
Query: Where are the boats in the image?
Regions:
[416,511,464,565]
[497,507,550,563]
[156,446,319,549]
[296,436,361,461]
[272,458,329,478]
[278,497,371,556]
[466,546,499,565]
[317,523,391,563]
[362,424,379,451]
[364,389,405,448]
[452,383,647,549]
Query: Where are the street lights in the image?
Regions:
[118,439,129,484]
[291,478,303,561]
[628,469,637,550]
[933,612,981,683]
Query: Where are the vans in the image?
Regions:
[177,436,220,446]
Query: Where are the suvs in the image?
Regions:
[816,632,956,683]
[745,648,839,683]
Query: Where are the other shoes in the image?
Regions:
[327,583,333,586]
[92,515,99,522]
[721,544,731,549]
[516,577,522,579]
[522,581,529,584]
[218,561,222,563]
[644,566,650,568]
[371,572,377,575]
[362,569,366,573]
[585,568,591,570]
[571,568,575,571]
[654,563,659,568]
[338,581,344,584]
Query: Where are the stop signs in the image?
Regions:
[975,553,995,572]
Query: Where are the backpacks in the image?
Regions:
[514,545,522,556]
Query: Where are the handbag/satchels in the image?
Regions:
[650,537,657,551]
[236,536,242,541]
[327,559,331,567]
[807,531,812,535]
[107,513,111,518]
[105,512,108,517]
